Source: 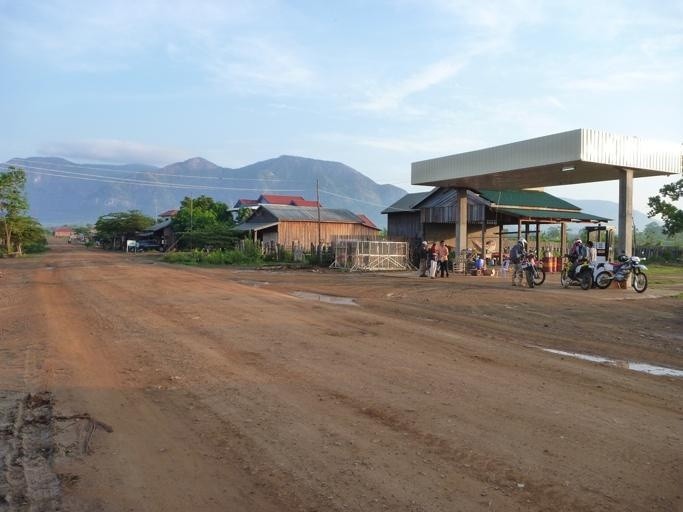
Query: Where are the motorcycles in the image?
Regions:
[520,251,546,290]
[560,255,650,292]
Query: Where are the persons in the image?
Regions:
[509,238,534,290]
[429,242,437,279]
[416,240,427,278]
[584,239,597,289]
[437,240,448,278]
[570,240,587,265]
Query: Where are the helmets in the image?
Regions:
[519,238,528,247]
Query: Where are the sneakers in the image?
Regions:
[511,283,526,287]
[419,273,450,279]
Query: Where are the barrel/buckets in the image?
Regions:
[476,257,485,269]
[543,257,557,272]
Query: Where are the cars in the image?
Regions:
[129,240,164,251]
